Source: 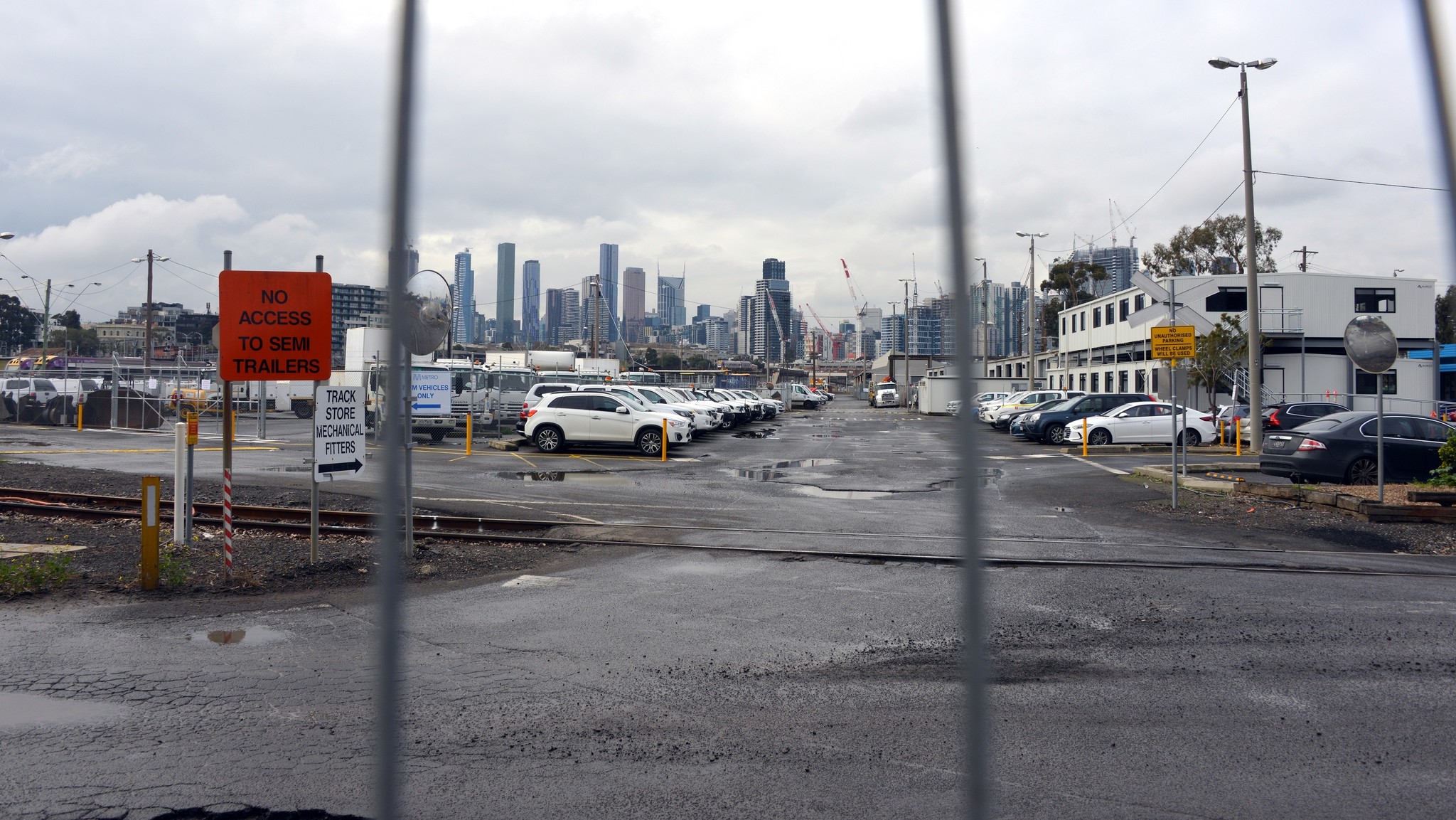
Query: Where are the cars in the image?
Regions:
[1201,401,1354,445]
[1259,411,1456,486]
[1063,401,1216,449]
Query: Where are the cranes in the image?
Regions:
[765,198,1137,374]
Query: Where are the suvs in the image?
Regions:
[946,391,1090,437]
[1019,394,1162,446]
[525,390,692,458]
[516,384,837,439]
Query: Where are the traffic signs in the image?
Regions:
[314,386,366,482]
[406,370,451,415]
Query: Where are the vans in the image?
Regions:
[49,379,101,408]
[0,379,59,408]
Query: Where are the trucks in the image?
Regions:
[196,356,662,443]
[868,381,900,409]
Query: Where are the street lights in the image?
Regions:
[898,278,916,387]
[887,301,901,384]
[1208,49,1279,454]
[1015,231,1050,392]
[974,257,987,378]
[590,274,603,358]
[805,331,822,387]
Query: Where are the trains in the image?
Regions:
[29,355,217,381]
[2,357,36,378]
